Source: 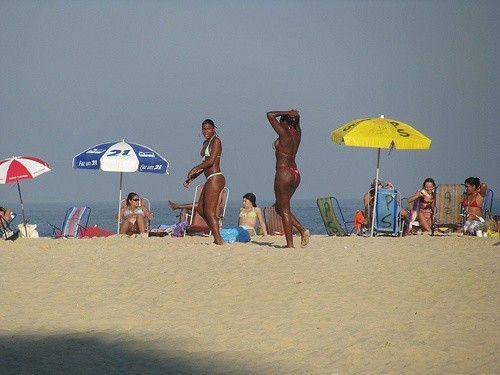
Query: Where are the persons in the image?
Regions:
[237,192,268,236]
[184,119,226,244]
[407,178,435,234]
[168,191,223,222]
[0,207,11,228]
[457,177,487,233]
[267,110,309,248]
[364,178,407,215]
[116,192,152,234]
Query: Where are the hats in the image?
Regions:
[371,178,389,188]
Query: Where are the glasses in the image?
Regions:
[132,199,139,201]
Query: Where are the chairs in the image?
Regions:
[264,207,284,235]
[317,197,356,236]
[120,198,151,238]
[183,184,229,237]
[47,206,91,239]
[364,184,494,237]
[0,213,17,237]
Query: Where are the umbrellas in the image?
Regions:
[72,139,169,235]
[331,115,432,236]
[0,156,51,237]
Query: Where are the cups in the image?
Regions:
[476,230,483,237]
